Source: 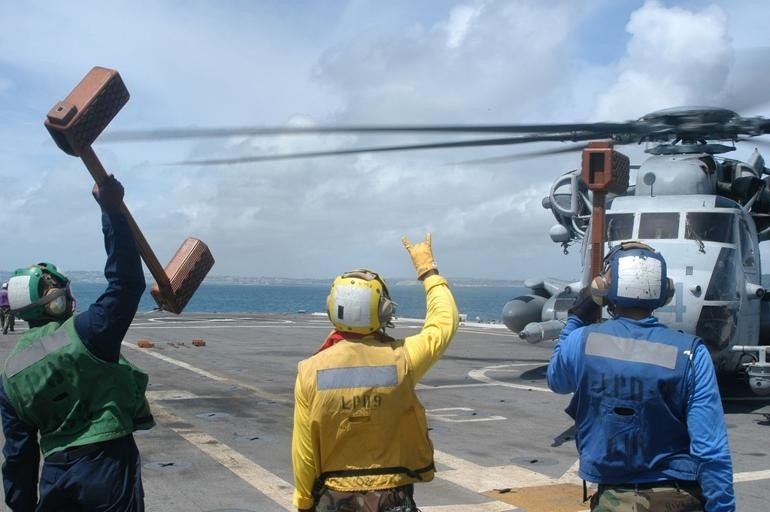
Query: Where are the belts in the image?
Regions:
[45,440,116,462]
[616,481,670,489]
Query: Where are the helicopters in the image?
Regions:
[91,44,770,413]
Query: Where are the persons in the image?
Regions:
[292,233,460,512]
[0,175,157,511]
[548,242,737,510]
[0,282,12,334]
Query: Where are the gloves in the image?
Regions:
[402,232,438,280]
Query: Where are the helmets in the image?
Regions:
[2,263,68,320]
[600,242,667,309]
[326,268,396,335]
